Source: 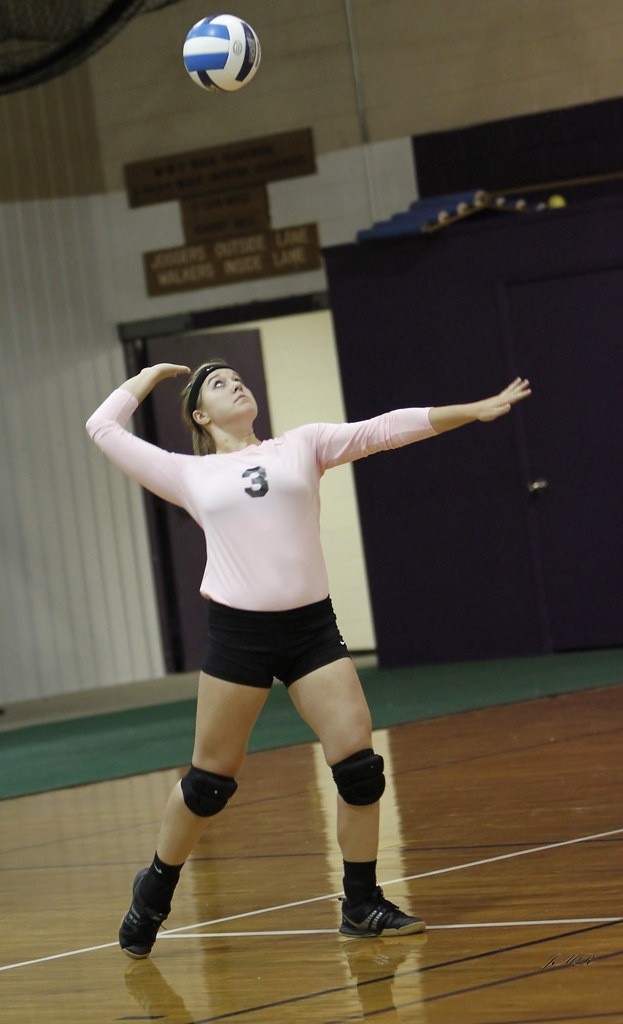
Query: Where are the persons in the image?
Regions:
[85,357,532,961]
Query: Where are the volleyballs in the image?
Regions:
[183,12,264,95]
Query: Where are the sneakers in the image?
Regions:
[343,934,427,972]
[124,958,183,1017]
[121,868,171,960]
[340,886,425,937]
